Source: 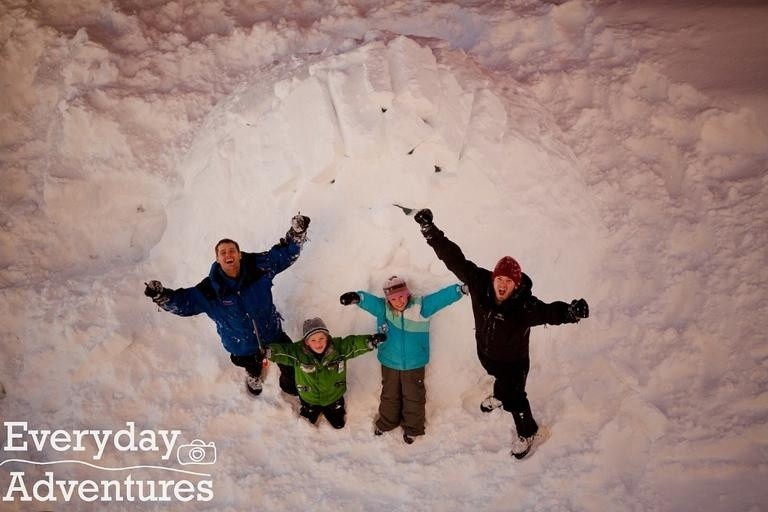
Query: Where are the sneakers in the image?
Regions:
[246,372,262,398]
[404,432,416,445]
[509,423,540,460]
[374,425,383,436]
[479,396,503,414]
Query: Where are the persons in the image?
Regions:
[257,317,388,430]
[412,207,591,461]
[140,210,311,397]
[336,274,471,446]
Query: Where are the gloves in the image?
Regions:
[144,279,170,306]
[340,291,360,306]
[413,208,434,228]
[373,333,387,346]
[461,283,469,295]
[291,215,311,235]
[572,298,589,319]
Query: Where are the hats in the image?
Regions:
[382,275,410,302]
[491,255,522,288]
[302,316,329,341]
